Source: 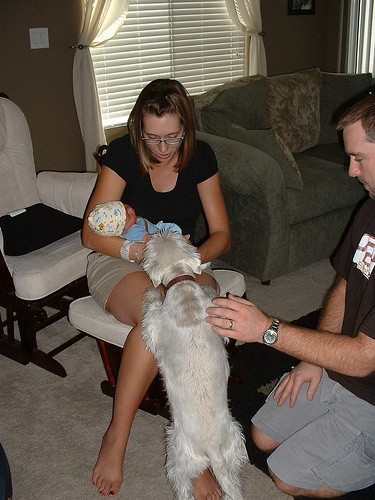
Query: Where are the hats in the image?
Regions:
[88,200,126,237]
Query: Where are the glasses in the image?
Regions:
[138,120,185,144]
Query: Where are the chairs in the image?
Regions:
[0,95,99,377]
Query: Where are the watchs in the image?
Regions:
[261,318,279,347]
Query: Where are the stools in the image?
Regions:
[68,268,246,423]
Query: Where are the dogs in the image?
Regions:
[140,232,250,500]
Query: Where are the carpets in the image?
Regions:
[160,306,375,500]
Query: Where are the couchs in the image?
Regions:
[192,73,375,285]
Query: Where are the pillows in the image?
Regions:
[196,74,270,139]
[265,65,321,154]
[319,71,372,144]
[229,121,304,191]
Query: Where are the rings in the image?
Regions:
[228,319,233,329]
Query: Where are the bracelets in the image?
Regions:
[120,240,135,262]
[135,240,144,264]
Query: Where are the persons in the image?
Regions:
[81,79,230,500]
[87,201,189,244]
[205,96,375,500]
